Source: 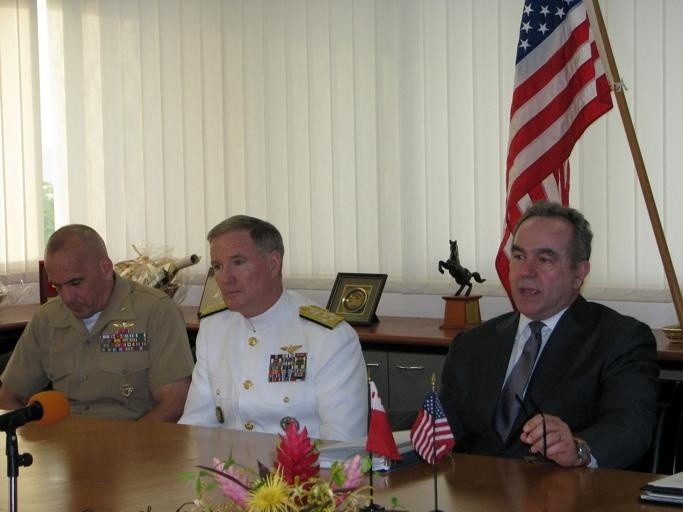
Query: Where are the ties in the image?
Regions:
[493,321,546,443]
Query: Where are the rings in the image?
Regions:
[554,431,563,442]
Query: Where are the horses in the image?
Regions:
[439,240,486,297]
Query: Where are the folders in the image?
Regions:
[314,426,419,474]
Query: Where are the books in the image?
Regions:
[639,471,683,509]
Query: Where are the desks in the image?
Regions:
[0,403,682,511]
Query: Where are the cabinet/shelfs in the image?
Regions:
[361,349,448,431]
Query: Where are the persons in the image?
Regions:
[172,213,369,444]
[434,200,661,474]
[0,222,194,425]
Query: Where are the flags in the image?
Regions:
[363,379,405,462]
[494,0,629,310]
[409,390,457,469]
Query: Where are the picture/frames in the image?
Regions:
[326,272,387,326]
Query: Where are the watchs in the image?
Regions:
[574,437,592,468]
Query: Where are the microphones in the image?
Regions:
[0,388,71,436]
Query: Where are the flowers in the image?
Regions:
[176,417,400,511]
